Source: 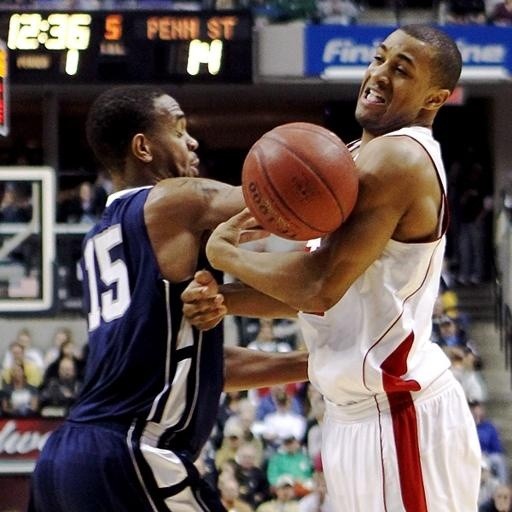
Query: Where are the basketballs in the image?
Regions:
[242,123,358,241]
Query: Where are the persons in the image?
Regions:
[182,23,481,512]
[27,83,309,511]
